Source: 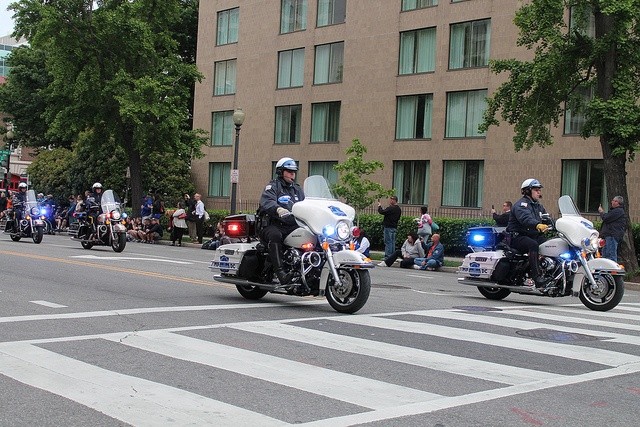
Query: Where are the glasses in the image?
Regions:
[532,187,541,192]
[96,187,102,189]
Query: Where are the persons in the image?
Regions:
[377,231,426,268]
[85,182,104,234]
[0,191,13,221]
[73,195,85,223]
[126,188,165,243]
[36,192,44,210]
[184,194,197,239]
[213,223,224,249]
[598,196,627,262]
[169,203,188,247]
[12,182,28,228]
[198,203,200,204]
[414,234,444,271]
[413,206,432,245]
[491,201,512,227]
[378,196,401,259]
[506,179,553,288]
[47,192,74,230]
[255,157,304,285]
[352,228,372,259]
[192,194,210,245]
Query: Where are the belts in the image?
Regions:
[255,214,275,224]
[506,227,520,232]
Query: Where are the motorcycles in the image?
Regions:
[456,195,626,311]
[208,174,375,314]
[2,188,46,243]
[68,188,128,252]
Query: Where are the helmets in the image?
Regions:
[18,182,28,191]
[275,157,298,179]
[520,178,544,198]
[92,182,103,193]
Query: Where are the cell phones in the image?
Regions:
[378,199,380,202]
[599,204,602,207]
[491,205,494,209]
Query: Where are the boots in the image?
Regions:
[528,250,553,287]
[269,241,293,285]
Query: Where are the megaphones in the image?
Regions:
[99,225,108,233]
[309,251,321,266]
[569,259,578,274]
[20,219,29,226]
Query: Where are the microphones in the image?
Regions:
[284,176,295,182]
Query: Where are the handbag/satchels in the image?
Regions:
[202,210,210,222]
[178,213,188,219]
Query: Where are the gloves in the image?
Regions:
[277,207,290,219]
[535,224,550,234]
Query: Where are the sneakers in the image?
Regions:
[377,260,387,266]
[414,264,422,269]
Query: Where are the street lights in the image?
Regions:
[5,129,14,202]
[231,107,245,212]
